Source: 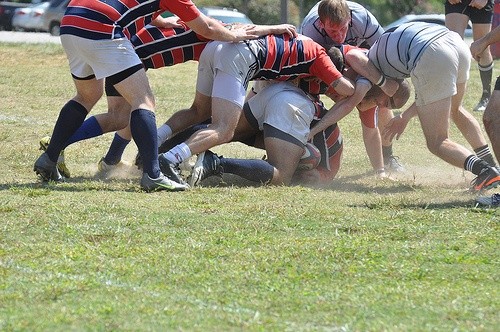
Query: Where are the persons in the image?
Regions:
[132,28,412,189]
[469,22,500,172]
[39,13,297,181]
[143,81,343,187]
[345,19,500,192]
[442,0,495,112]
[293,0,401,176]
[136,81,344,188]
[33,0,259,192]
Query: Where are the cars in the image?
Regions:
[0,0,72,36]
[201,6,254,26]
[383,14,473,37]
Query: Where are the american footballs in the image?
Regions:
[294,143,322,176]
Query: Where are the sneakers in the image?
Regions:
[382,154,404,173]
[462,165,500,196]
[135,151,143,167]
[39,136,70,178]
[190,149,225,188]
[158,153,192,189]
[139,171,186,193]
[33,153,66,184]
[94,157,143,180]
[474,193,500,207]
[365,168,385,178]
[473,97,490,111]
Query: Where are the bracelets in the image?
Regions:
[376,75,387,88]
